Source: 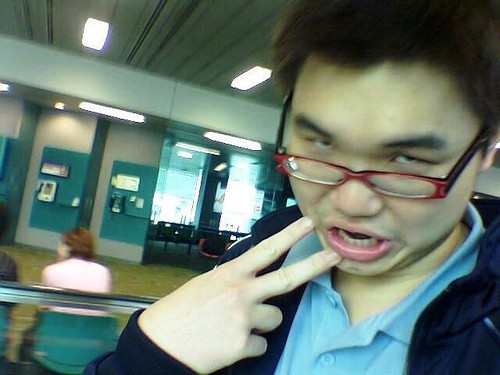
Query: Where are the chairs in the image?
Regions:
[156,221,251,255]
[20,310,118,375]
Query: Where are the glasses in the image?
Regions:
[273,89,490,200]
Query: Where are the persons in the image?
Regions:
[84,0,500,375]
[17,228,111,361]
[0,252,20,308]
